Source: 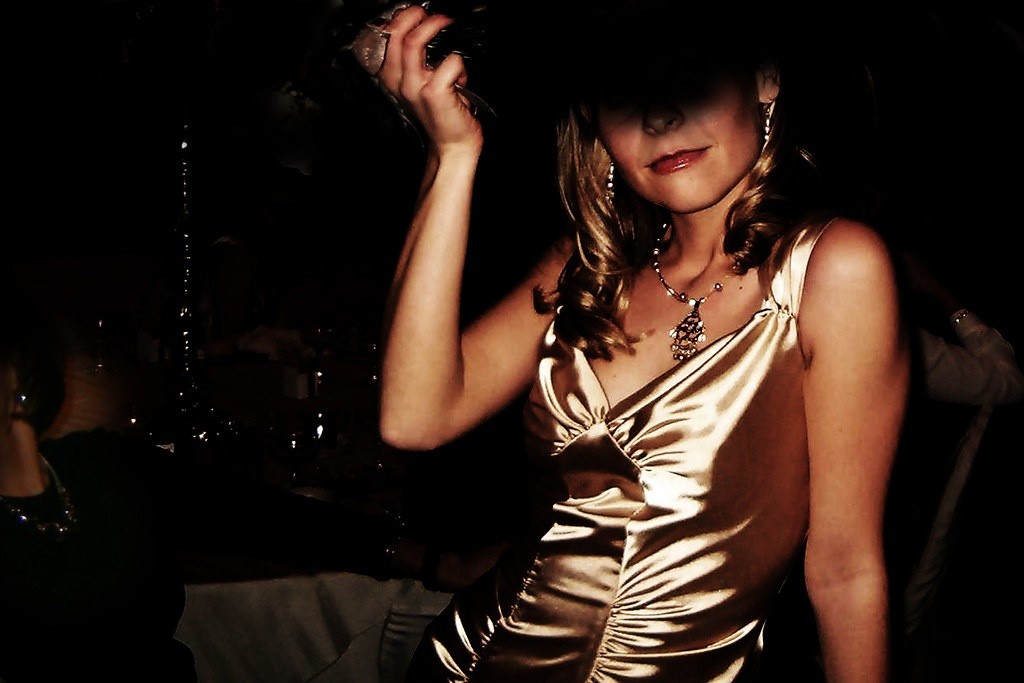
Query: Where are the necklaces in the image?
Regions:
[651,217,743,363]
[1,447,82,541]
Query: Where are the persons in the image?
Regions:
[0,356,224,683]
[159,0,913,683]
[892,252,1023,408]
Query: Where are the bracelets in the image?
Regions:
[951,310,969,328]
